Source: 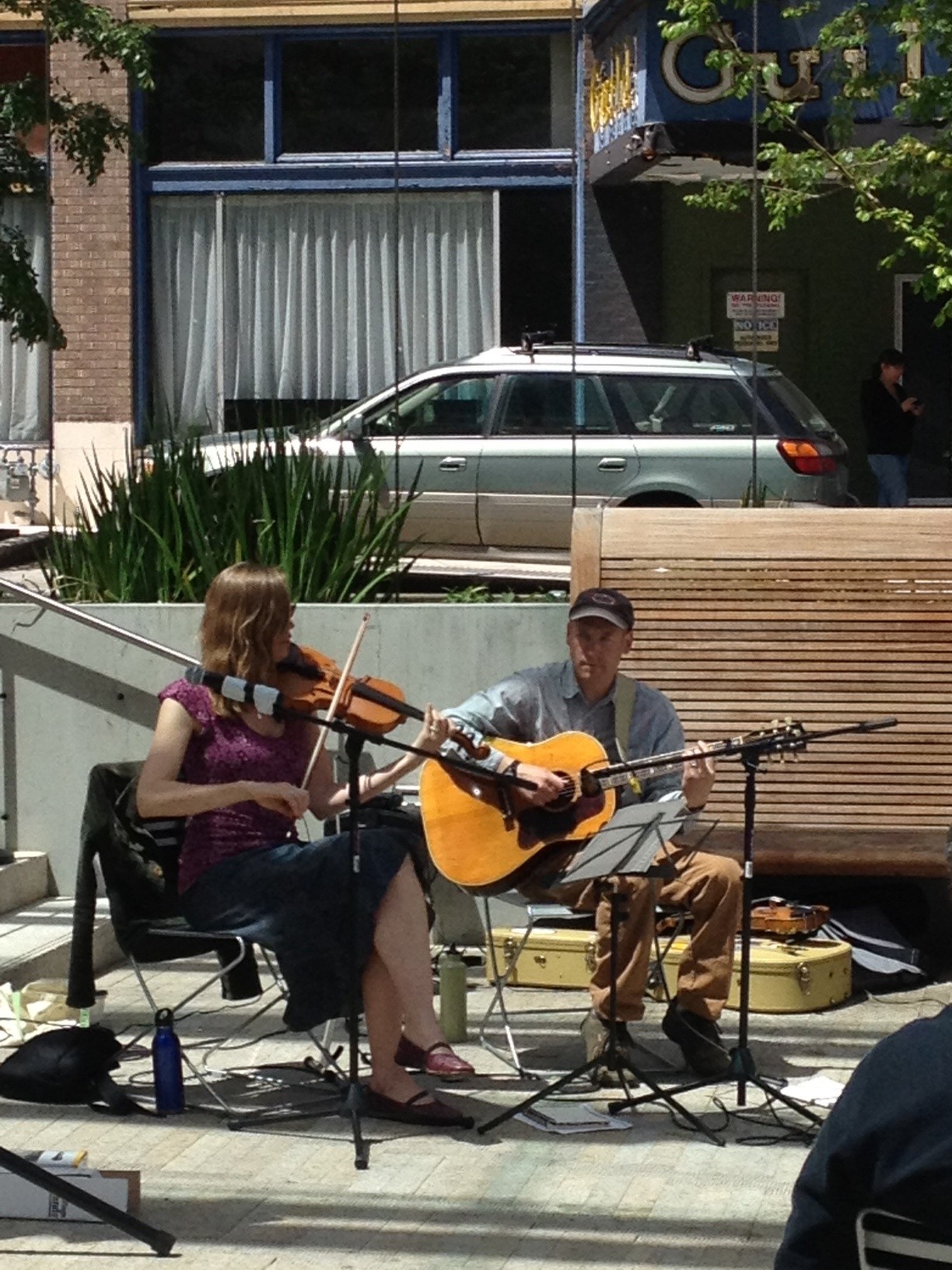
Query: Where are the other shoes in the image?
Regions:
[394,1031,475,1075]
[363,1085,463,1124]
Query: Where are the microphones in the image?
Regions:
[578,767,602,798]
[181,668,284,708]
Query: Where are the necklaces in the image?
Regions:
[246,703,264,721]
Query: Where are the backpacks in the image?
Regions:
[0,1023,134,1116]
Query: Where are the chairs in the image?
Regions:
[479,896,694,1076]
[67,761,350,1119]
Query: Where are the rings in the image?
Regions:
[430,725,440,732]
[691,764,698,767]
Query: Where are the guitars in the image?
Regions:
[419,716,808,896]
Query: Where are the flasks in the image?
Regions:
[151,1007,185,1116]
[439,942,468,1043]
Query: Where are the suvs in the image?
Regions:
[137,328,852,550]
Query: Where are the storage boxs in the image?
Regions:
[0,1148,141,1222]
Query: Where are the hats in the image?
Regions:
[569,587,634,631]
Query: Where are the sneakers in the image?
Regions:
[580,1007,638,1088]
[661,995,736,1081]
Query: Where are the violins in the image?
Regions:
[657,897,828,934]
[271,644,491,761]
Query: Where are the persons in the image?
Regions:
[773,1004,952,1270]
[136,561,475,1124]
[861,349,926,507]
[440,589,743,1087]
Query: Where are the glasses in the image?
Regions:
[289,606,296,618]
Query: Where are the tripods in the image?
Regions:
[224,691,543,1170]
[475,814,726,1146]
[597,719,902,1127]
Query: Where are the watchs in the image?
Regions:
[502,758,522,787]
